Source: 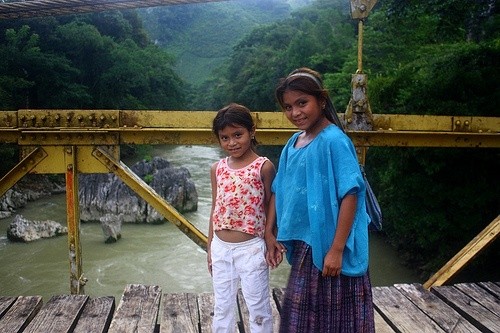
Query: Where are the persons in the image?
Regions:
[266,67,382,333]
[207,103,287,333]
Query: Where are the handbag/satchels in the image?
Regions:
[359,163,384,232]
